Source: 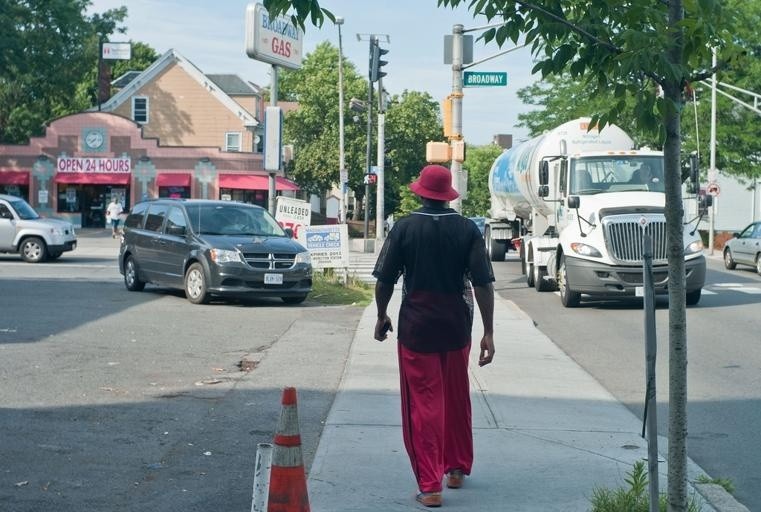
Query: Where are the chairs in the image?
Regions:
[573,171,594,190]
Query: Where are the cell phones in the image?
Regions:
[379,321,391,338]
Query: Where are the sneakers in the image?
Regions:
[447,469,464,488]
[417,490,443,506]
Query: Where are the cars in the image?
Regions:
[722,220,761,273]
[469,216,488,237]
[0,193,80,265]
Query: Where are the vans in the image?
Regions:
[116,193,315,306]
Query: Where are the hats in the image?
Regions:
[409,165,458,200]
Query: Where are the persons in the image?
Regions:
[105,195,125,239]
[217,214,251,233]
[369,162,499,507]
[626,162,661,192]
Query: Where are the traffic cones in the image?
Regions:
[266,385,313,511]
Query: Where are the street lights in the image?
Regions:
[332,15,349,224]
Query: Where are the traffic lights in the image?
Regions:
[372,44,391,83]
[364,173,378,185]
